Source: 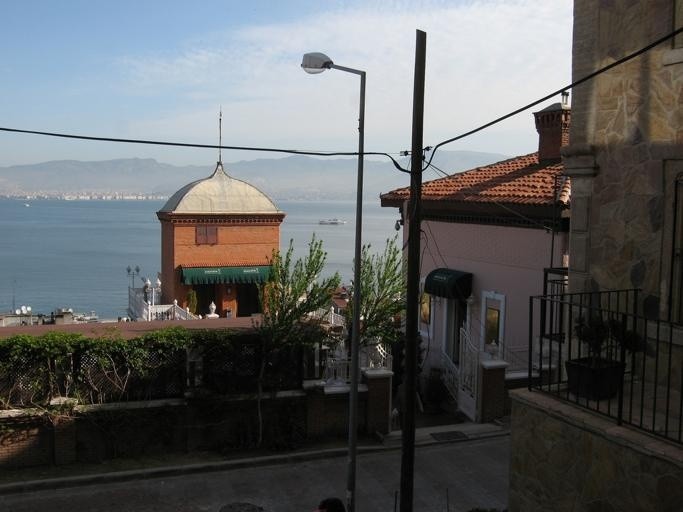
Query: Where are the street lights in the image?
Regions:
[127,265,140,290]
[16,306,32,326]
[301,52,365,510]
[145,277,161,305]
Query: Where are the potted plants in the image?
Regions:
[566,314,647,402]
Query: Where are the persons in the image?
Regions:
[316,497,346,512]
